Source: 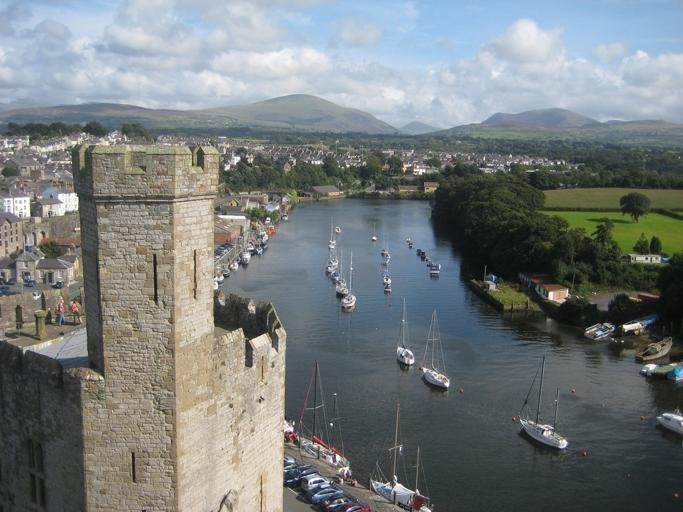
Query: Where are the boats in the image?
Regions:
[402,230,442,281]
[213,212,290,293]
[654,408,683,436]
[640,360,683,385]
[582,321,615,342]
[618,309,660,336]
[635,336,673,360]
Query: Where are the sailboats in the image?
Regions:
[370,224,397,295]
[324,214,359,312]
[370,403,435,512]
[518,353,569,450]
[394,297,414,366]
[419,308,451,391]
[296,360,352,479]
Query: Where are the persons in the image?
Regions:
[55,296,85,325]
[335,464,359,487]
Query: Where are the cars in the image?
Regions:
[284,453,371,512]
[27,279,37,288]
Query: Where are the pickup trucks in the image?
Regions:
[52,280,64,288]
